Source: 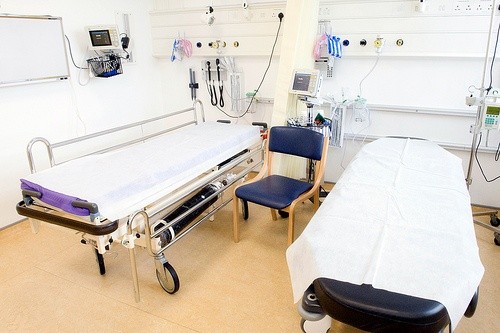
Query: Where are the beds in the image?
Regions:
[15,99,268,303]
[284,133,486,333]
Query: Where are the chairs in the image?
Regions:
[233,125,329,243]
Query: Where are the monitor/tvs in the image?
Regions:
[84,24,119,48]
[288,68,321,96]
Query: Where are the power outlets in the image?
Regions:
[450,2,500,13]
[270,8,285,24]
